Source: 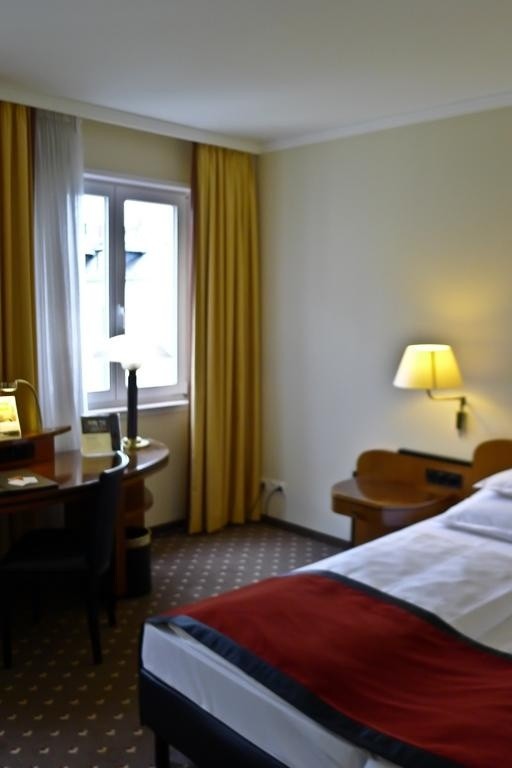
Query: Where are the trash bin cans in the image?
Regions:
[122,526,151,598]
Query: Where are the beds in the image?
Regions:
[138,439,512,768]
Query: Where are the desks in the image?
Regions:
[0,426,170,603]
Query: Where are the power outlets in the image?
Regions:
[260,478,286,498]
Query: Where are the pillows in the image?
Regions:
[472,467,512,500]
[443,489,512,543]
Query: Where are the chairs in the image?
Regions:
[0,451,130,669]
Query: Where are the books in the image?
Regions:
[1,467,59,494]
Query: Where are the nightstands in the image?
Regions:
[332,449,472,547]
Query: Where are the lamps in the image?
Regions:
[92,333,170,468]
[391,344,466,429]
[0,378,44,433]
